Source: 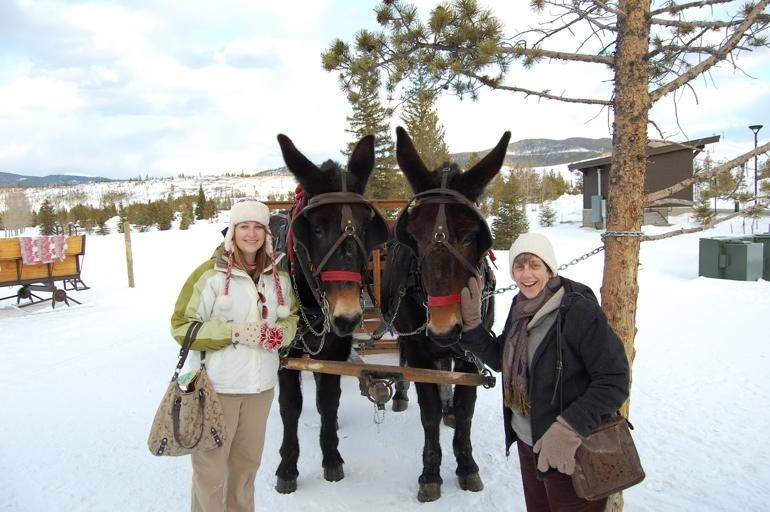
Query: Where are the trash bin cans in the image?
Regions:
[117,221,124,234]
[735,201,740,212]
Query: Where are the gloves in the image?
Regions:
[532,415,583,477]
[232,319,285,354]
[458,276,486,335]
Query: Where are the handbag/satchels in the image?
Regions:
[146,319,229,459]
[567,412,647,502]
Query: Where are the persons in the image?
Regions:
[457,233,631,512]
[168,201,300,512]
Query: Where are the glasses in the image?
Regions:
[255,290,270,321]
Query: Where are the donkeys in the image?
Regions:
[268,133,390,495]
[379,125,512,503]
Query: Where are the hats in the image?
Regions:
[507,231,559,283]
[224,196,275,259]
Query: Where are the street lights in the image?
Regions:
[749,125,762,205]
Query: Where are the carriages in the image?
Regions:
[260,126,515,503]
